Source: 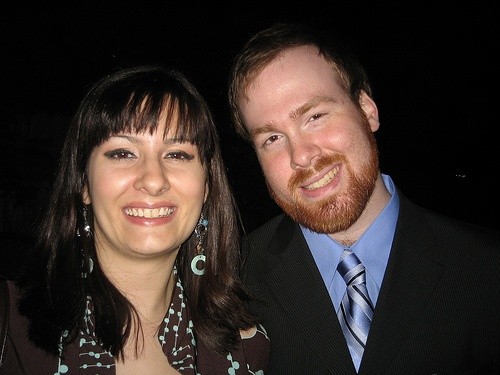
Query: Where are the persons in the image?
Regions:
[0,66,272,375]
[228,25,500,375]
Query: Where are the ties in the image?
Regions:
[332,250,373,373]
